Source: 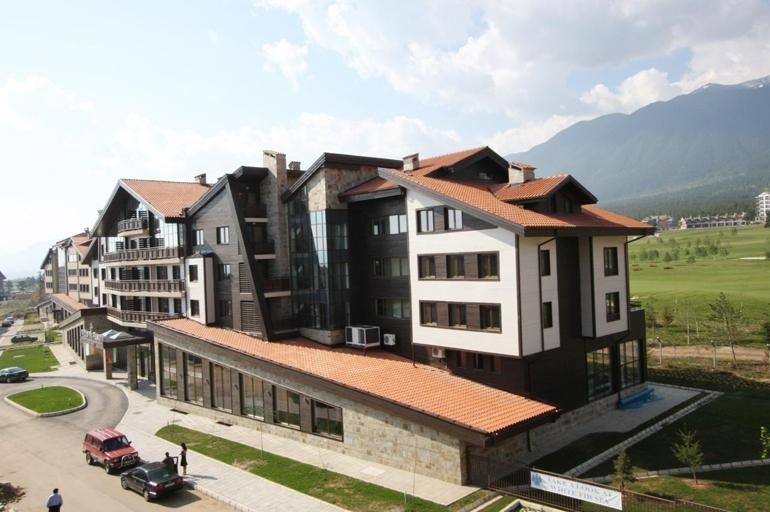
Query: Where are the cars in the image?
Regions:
[11,334,38,343]
[119,455,183,502]
[5,317,14,324]
[2,320,12,327]
[0,366,30,383]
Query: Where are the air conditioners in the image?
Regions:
[431,346,446,359]
[384,333,396,346]
[345,324,381,357]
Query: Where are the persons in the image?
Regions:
[46,487,64,511]
[179,441,190,475]
[163,451,175,464]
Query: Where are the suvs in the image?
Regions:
[82,428,141,474]
